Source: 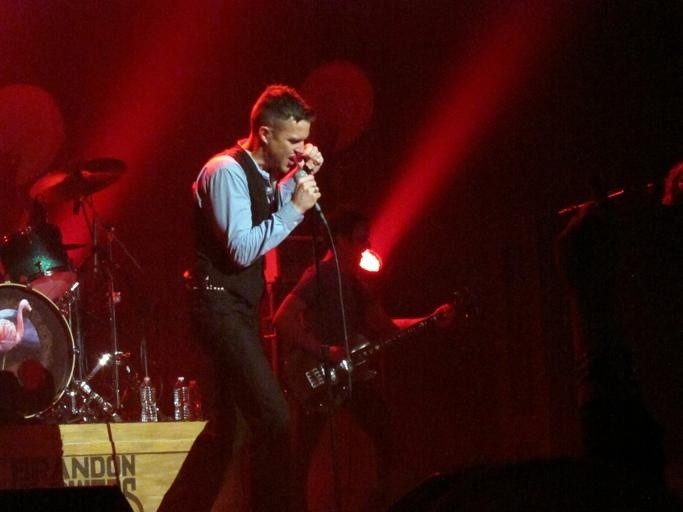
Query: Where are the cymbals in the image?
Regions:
[29,158,126,204]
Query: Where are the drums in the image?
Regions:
[1,224,80,302]
[1,281,77,423]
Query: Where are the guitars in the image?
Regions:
[283,273,501,415]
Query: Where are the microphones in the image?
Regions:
[295,169,321,214]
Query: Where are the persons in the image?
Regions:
[271,201,457,512]
[187,84,317,512]
[553,150,682,502]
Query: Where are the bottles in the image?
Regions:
[174,376,190,421]
[140,376,159,422]
